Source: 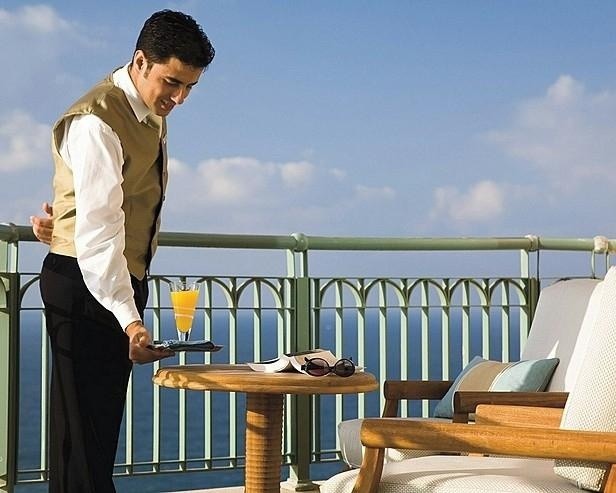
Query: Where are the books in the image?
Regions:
[247,348,362,377]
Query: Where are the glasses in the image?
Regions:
[301,355,358,378]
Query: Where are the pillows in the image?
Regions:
[433,355,559,415]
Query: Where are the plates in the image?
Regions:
[152,339,224,354]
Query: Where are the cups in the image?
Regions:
[170,281,202,341]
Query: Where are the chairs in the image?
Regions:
[338,278,601,469]
[319,266,616,492]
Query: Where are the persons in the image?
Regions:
[30,10,215,493]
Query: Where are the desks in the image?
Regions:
[152,365,378,492]
